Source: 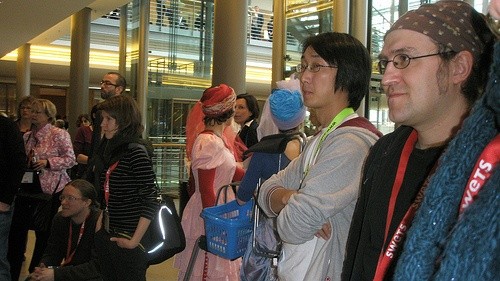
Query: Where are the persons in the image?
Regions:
[172,83,247,281]
[154,0,275,43]
[23,179,118,281]
[234,89,309,281]
[233,92,262,203]
[93,94,163,281]
[309,109,325,135]
[84,71,128,210]
[22,98,77,273]
[1,96,102,181]
[340,0,500,281]
[257,31,385,280]
[0,113,27,281]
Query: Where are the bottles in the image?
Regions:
[31,145,41,175]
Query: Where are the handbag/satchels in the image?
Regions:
[199,182,253,262]
[21,167,54,208]
[139,196,186,266]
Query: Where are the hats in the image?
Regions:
[270,87,306,132]
[385,1,492,89]
[197,84,237,115]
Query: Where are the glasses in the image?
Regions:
[376,50,458,72]
[80,120,88,124]
[294,63,339,71]
[100,81,118,88]
[57,194,83,201]
